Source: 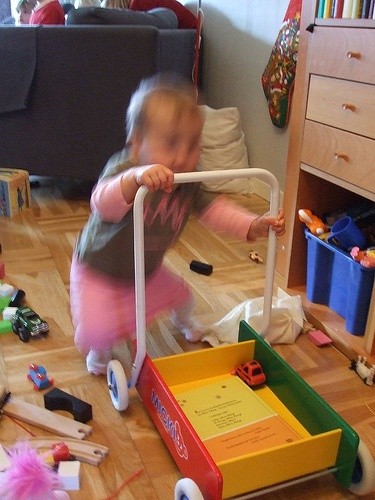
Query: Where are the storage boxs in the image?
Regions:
[304,227,375,336]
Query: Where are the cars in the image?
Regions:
[236,360,266,386]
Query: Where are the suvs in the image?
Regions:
[10,306,49,343]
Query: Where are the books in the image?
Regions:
[317,0,375,19]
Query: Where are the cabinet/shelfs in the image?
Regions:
[284,0,375,202]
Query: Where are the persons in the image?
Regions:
[16,0,198,29]
[70,73,286,377]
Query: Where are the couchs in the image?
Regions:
[0,25,199,182]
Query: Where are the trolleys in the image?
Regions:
[106,168,375,500]
